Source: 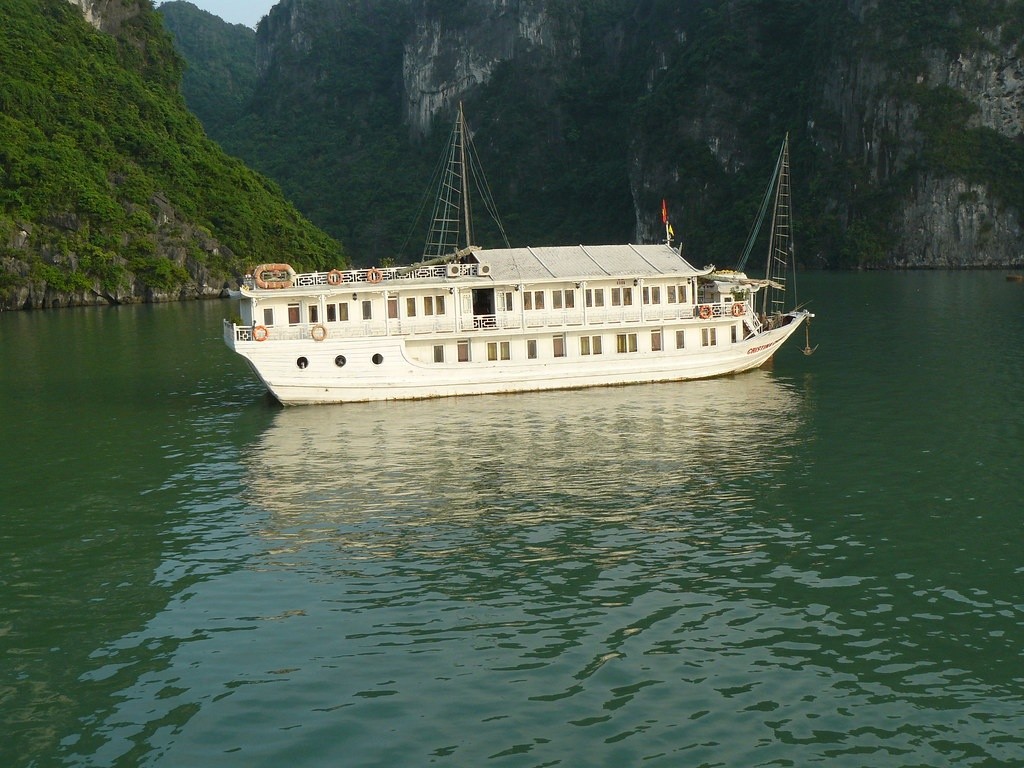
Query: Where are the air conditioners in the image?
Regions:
[446,264,461,277]
[477,263,492,276]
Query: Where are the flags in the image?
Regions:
[662,200,666,223]
[669,224,674,236]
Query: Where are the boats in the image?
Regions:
[213,101,818,408]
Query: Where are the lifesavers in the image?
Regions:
[732,303,743,317]
[368,269,382,283]
[700,305,712,318]
[254,263,297,288]
[253,325,269,341]
[312,324,327,341]
[327,270,342,285]
